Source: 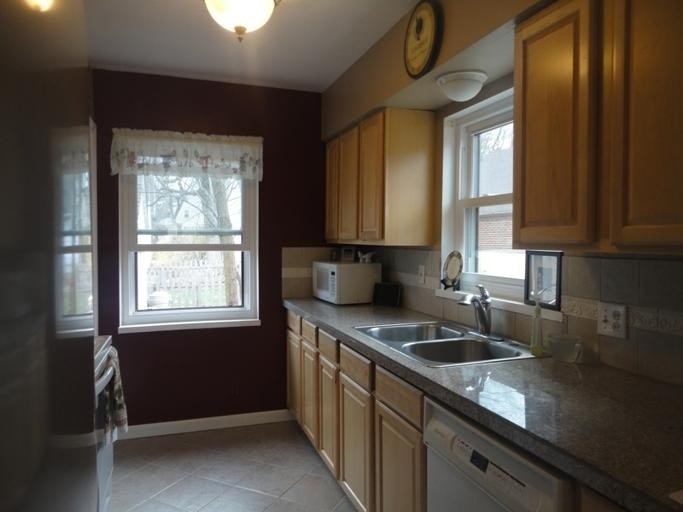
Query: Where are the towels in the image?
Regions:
[106,358,128,443]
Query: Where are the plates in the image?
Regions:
[443,250,463,286]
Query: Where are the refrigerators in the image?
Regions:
[419,392,571,509]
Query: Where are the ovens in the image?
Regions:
[94,335,118,512]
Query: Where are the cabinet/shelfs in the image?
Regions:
[324,108,437,248]
[285,311,425,512]
[512,0,683,251]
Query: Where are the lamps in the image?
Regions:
[204,0,280,42]
[436,72,488,104]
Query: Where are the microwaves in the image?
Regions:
[312,260,382,305]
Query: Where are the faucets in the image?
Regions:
[457,284,492,336]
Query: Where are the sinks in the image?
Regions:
[351,320,464,342]
[395,339,553,367]
